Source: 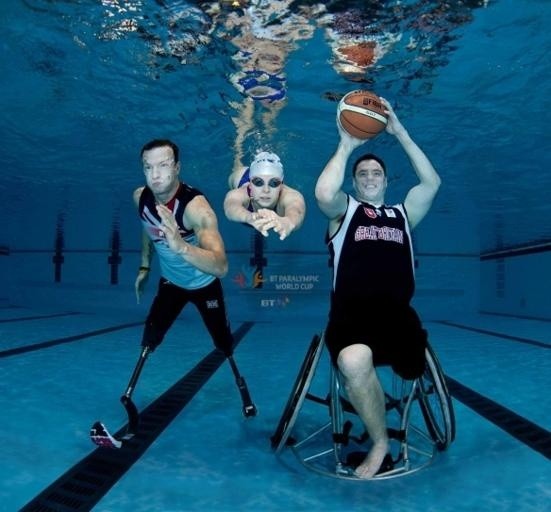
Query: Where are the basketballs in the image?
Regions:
[337,90,388,139]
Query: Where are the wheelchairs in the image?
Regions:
[269,309,464,482]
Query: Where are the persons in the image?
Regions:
[315,95,444,481]
[130,138,235,361]
[222,149,311,242]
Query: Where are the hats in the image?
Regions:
[248,151,284,182]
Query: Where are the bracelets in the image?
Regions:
[138,265,151,274]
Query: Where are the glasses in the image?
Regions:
[251,177,282,188]
